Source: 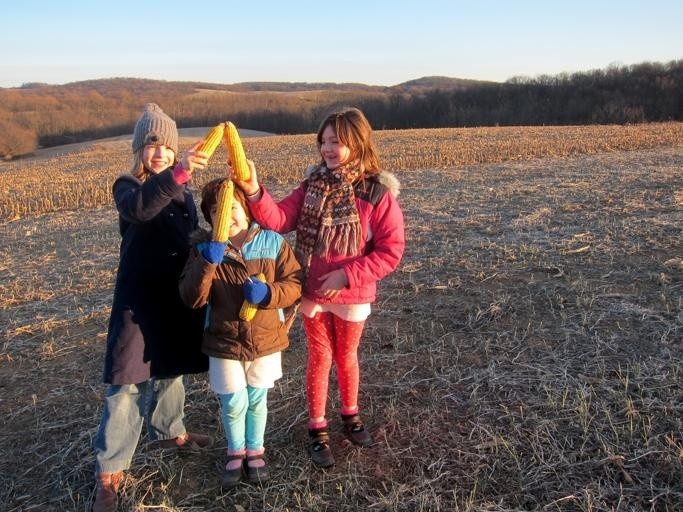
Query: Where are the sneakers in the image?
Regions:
[160,432,214,450]
[92,470,125,512]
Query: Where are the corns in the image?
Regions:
[211,179,234,243]
[196,122,225,162]
[224,121,251,182]
[239,273,266,321]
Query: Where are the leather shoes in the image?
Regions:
[343,416,372,447]
[309,434,335,469]
[246,452,270,484]
[222,454,246,488]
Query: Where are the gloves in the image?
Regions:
[198,241,227,266]
[244,277,270,306]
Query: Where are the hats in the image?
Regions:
[132,102,179,152]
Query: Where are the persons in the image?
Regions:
[228,105,406,468]
[179,177,304,488]
[92,100,209,511]
